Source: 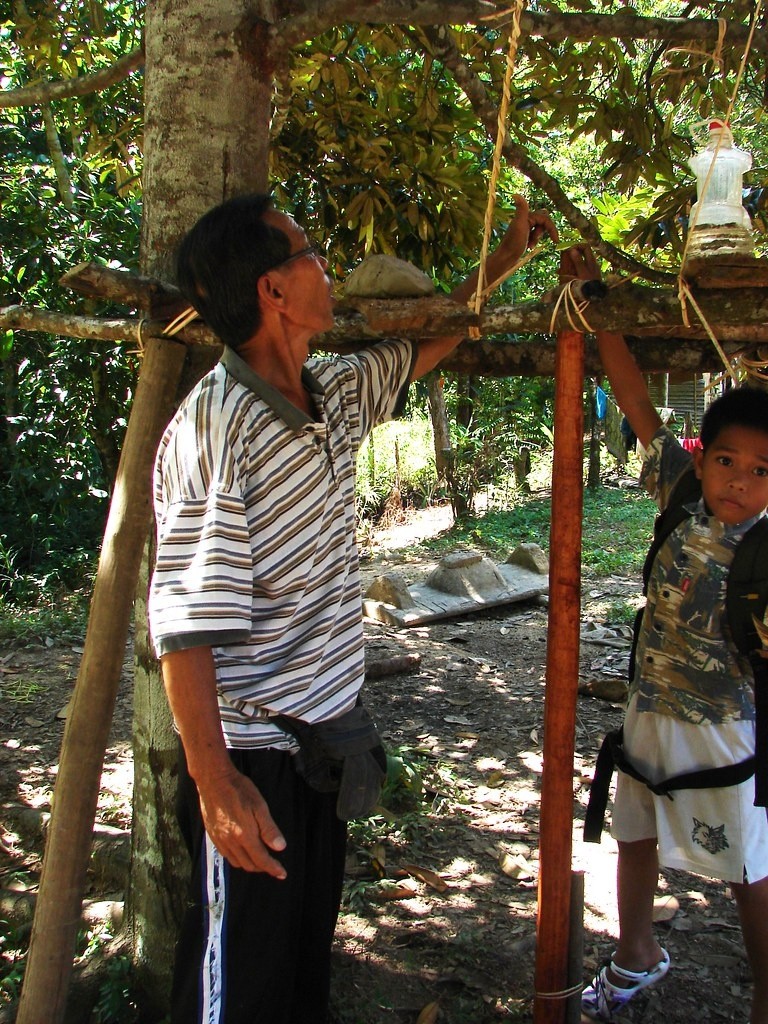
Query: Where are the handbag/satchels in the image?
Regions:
[270,694,388,822]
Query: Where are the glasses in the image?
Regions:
[261,239,323,280]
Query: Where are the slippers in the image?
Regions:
[581,946,670,1018]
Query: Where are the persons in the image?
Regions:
[147,195,559,1024]
[559,242,768,1024]
